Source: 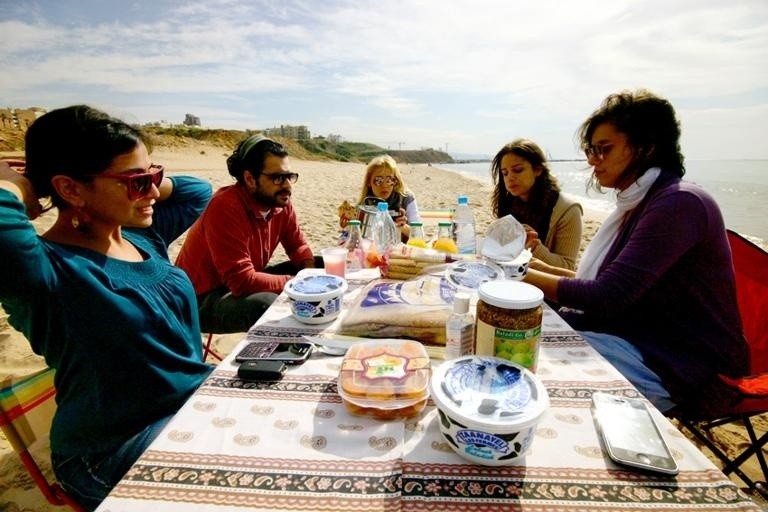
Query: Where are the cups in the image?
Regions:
[320,247,348,278]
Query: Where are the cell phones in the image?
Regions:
[591,393,680,478]
[234,342,313,365]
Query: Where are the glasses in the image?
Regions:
[93,166,166,197]
[586,141,619,159]
[371,175,398,188]
[253,172,299,185]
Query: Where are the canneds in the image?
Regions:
[474,279,544,375]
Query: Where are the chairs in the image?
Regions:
[645,229,768,503]
[0,368,89,512]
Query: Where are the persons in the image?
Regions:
[0,105,216,505]
[356,156,420,244]
[491,140,583,270]
[175,133,325,334]
[521,94,748,419]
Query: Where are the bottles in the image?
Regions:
[407,221,428,250]
[445,292,474,360]
[366,202,397,257]
[433,221,457,255]
[453,195,477,255]
[345,220,364,274]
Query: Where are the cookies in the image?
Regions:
[366,243,463,281]
